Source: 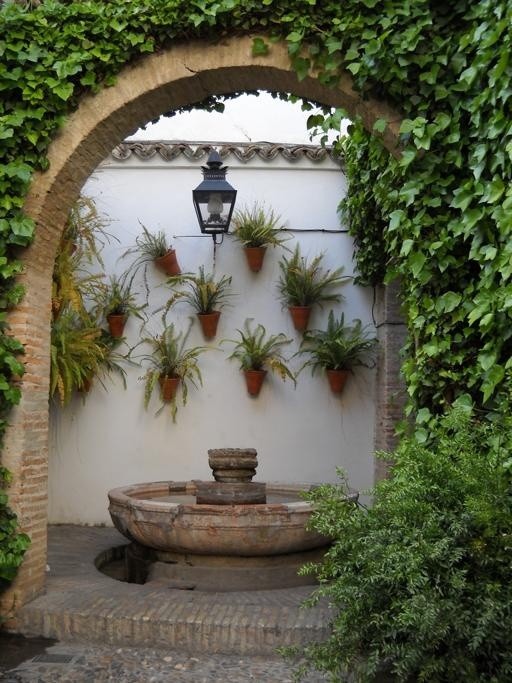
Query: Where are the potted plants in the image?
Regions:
[156,266,231,336]
[59,192,122,270]
[274,244,349,331]
[69,303,127,392]
[219,318,296,396]
[290,309,379,393]
[132,326,199,424]
[115,222,181,276]
[83,277,147,337]
[226,202,291,272]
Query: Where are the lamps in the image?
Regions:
[193,148,238,246]
